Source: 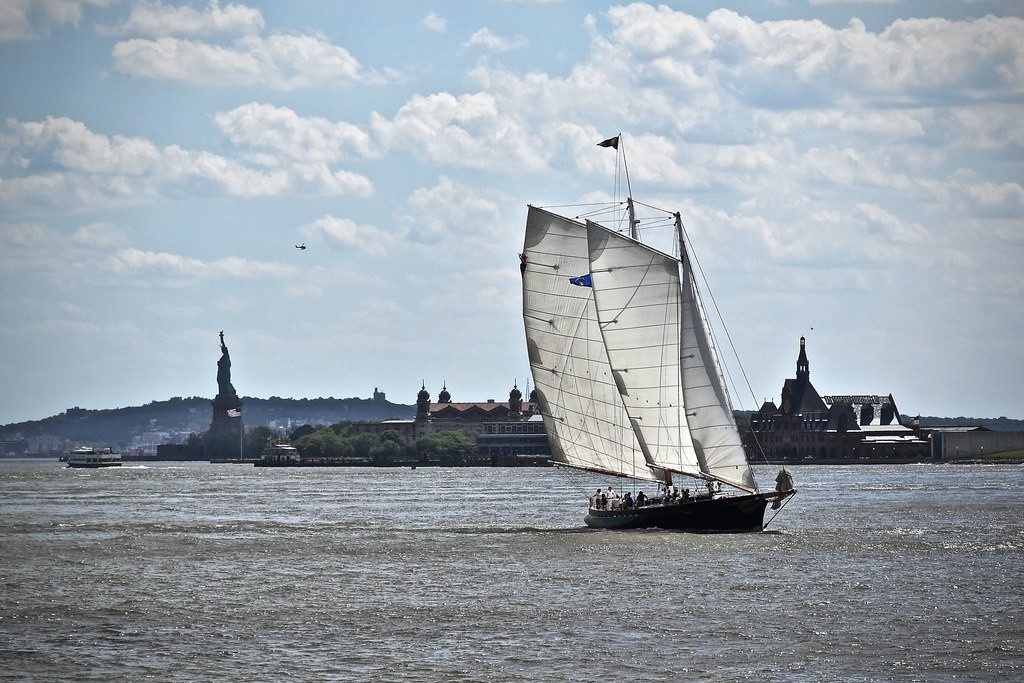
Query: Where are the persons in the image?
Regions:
[592,486,691,510]
[217,331,236,395]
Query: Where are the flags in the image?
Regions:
[569,274,592,287]
[227,406,241,417]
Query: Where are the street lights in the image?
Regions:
[981,447,983,460]
[956,446,958,459]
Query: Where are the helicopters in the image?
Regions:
[295,242,306,250]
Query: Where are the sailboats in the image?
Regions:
[518,198,797,533]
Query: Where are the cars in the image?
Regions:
[805,455,816,459]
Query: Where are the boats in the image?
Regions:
[67,446,122,468]
[261,445,300,460]
